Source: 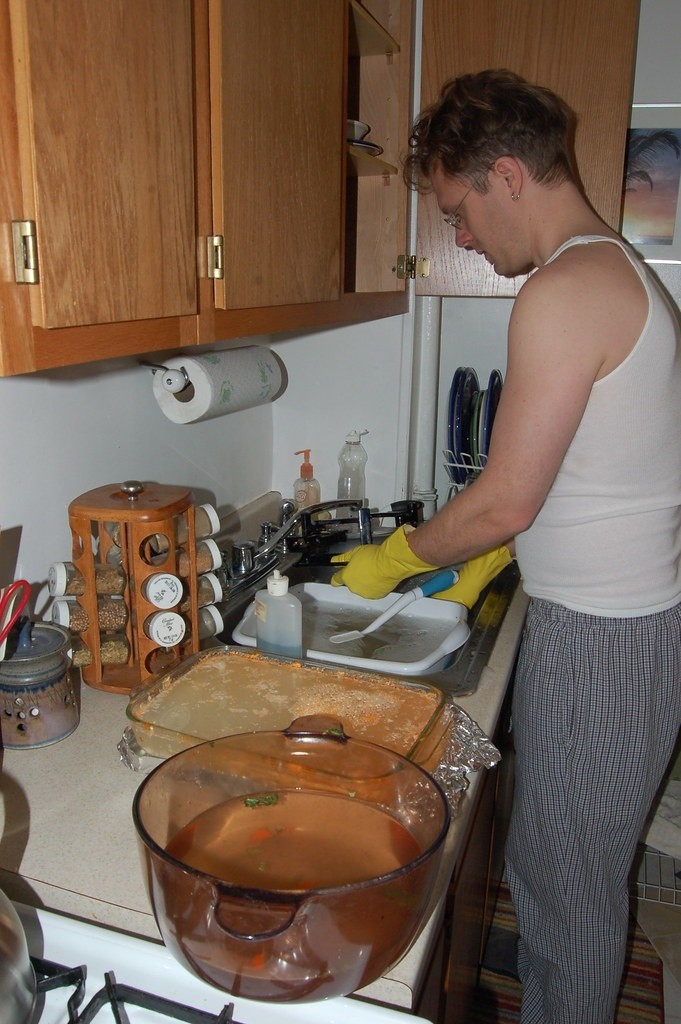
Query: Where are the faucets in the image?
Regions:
[212,499,364,603]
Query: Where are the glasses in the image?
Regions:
[444,155,514,230]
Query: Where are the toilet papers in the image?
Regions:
[152,344,282,425]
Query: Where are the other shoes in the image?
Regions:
[481,926,522,984]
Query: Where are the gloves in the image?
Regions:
[430,545,512,609]
[331,524,441,600]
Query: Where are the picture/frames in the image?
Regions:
[617,104,681,265]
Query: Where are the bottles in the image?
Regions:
[334,428,369,534]
[254,570,303,659]
[46,501,223,669]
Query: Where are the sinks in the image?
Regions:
[197,532,522,698]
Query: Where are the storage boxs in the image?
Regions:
[231,581,473,676]
[122,645,455,802]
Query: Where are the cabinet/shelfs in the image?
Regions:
[412,644,522,1024]
[0,1,640,381]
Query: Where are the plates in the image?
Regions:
[446,365,503,483]
[347,139,382,157]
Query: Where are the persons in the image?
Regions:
[330,66,681,1024]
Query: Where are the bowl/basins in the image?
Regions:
[131,714,451,1006]
[346,119,369,140]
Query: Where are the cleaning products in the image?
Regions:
[335,428,370,529]
[292,448,322,539]
[253,568,302,660]
